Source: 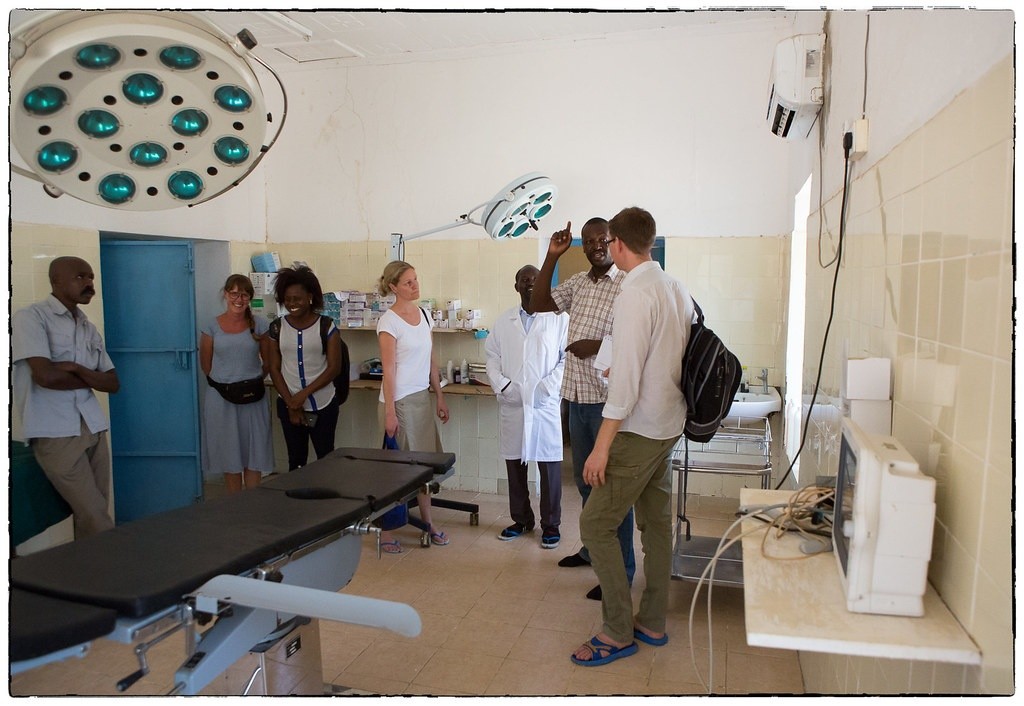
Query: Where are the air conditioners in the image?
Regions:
[765,33,826,144]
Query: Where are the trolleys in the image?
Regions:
[672,415,773,589]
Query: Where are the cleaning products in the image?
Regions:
[740,365,750,393]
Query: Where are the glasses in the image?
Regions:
[605,239,615,246]
[226,289,250,301]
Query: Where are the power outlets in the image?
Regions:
[847,119,869,163]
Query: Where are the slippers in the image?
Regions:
[571,634,638,666]
[498,523,529,540]
[432,532,449,544]
[383,541,403,554]
[635,630,668,645]
[541,533,559,548]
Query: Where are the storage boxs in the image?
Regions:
[251,252,481,331]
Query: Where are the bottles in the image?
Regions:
[446,359,469,384]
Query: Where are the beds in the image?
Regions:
[9,445,457,697]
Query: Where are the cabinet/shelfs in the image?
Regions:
[334,325,495,398]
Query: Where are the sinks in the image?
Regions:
[721,386,782,426]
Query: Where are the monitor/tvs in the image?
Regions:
[829,417,936,617]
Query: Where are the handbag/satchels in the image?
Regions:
[681,298,742,442]
[373,436,408,529]
[206,375,265,404]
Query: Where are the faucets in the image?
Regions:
[754,368,770,395]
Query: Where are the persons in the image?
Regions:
[484,264,570,548]
[376,261,449,554]
[571,207,698,666]
[268,265,342,471]
[10,256,120,541]
[200,274,274,493]
[529,218,635,600]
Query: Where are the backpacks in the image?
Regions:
[273,316,350,405]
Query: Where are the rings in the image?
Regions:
[593,475,598,477]
